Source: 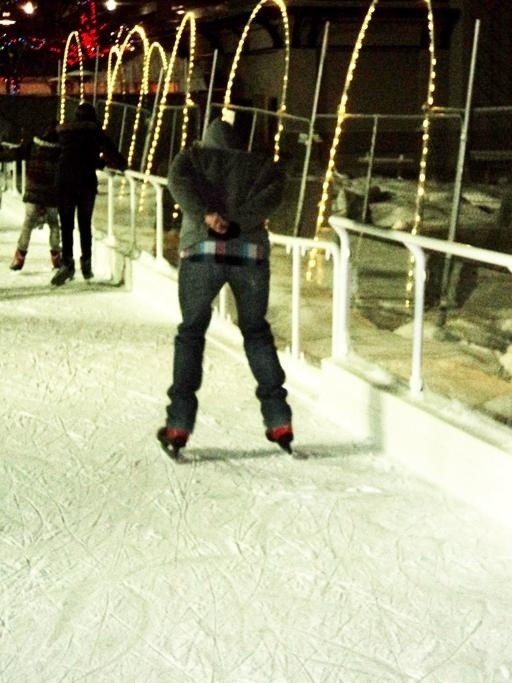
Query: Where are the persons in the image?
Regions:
[0,104,64,271]
[49,104,129,285]
[157,117,294,446]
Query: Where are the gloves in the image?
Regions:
[208,221,243,242]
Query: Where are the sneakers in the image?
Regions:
[10,250,27,268]
[50,262,75,285]
[157,424,191,448]
[82,267,94,279]
[263,425,293,445]
[50,249,64,268]
